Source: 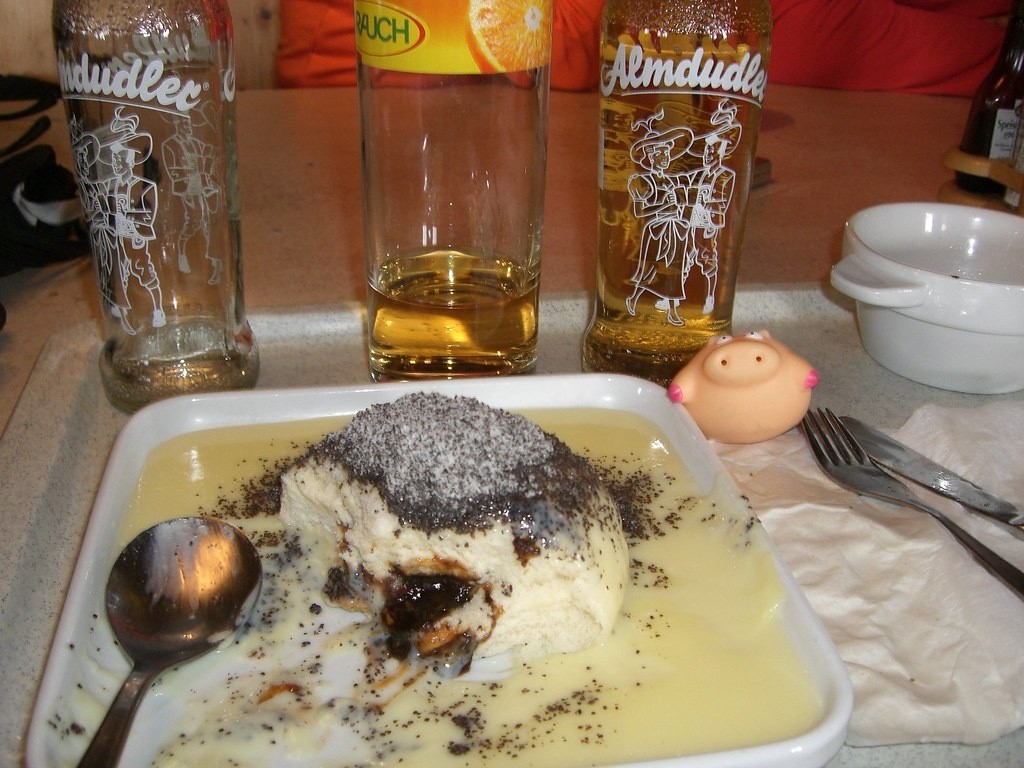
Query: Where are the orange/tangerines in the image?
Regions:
[468,2,552,75]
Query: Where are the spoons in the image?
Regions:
[77,514,264,768]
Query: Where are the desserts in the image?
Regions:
[280,394,631,678]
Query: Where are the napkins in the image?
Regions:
[693,399,1024,747]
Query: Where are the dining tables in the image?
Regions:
[0,84,1024,440]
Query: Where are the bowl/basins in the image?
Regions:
[829,202,1024,395]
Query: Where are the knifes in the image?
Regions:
[839,415,1024,532]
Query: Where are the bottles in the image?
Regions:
[351,1,550,381]
[54,1,262,410]
[583,1,771,386]
[954,0,1024,193]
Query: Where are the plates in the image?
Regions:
[22,372,853,768]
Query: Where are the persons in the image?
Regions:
[275,0,1007,96]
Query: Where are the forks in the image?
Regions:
[803,407,1024,601]
